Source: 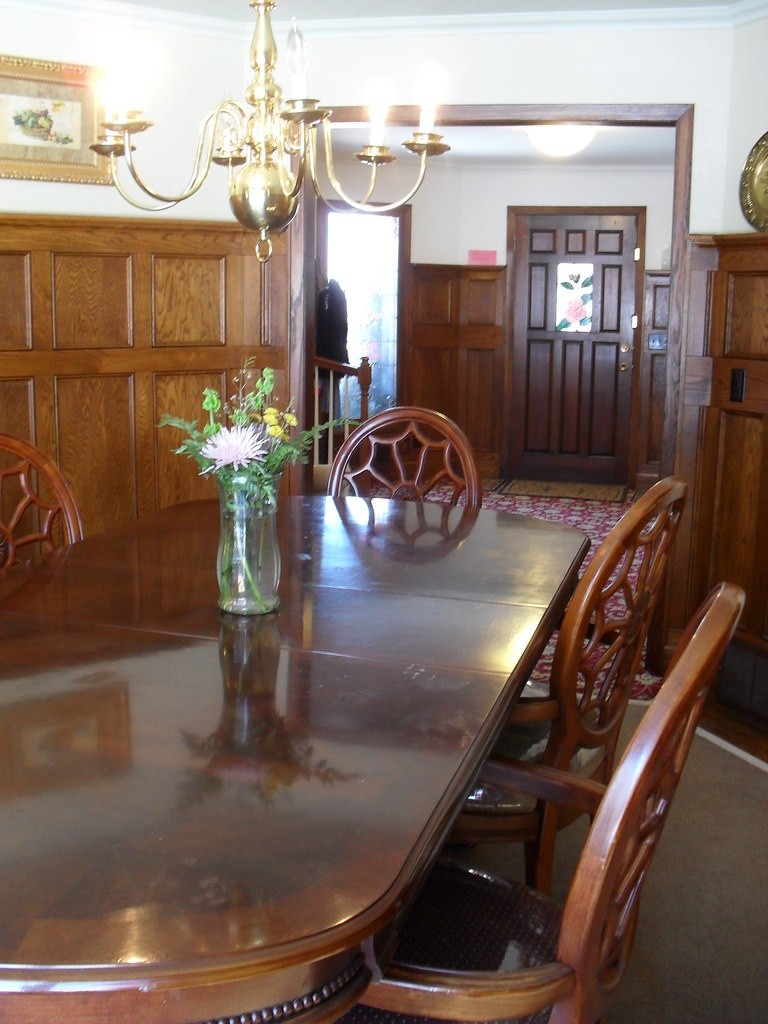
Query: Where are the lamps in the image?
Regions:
[90,0,453,273]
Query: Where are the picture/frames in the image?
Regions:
[0,48,116,190]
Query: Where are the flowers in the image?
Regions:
[164,363,351,491]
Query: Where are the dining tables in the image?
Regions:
[0,487,589,1020]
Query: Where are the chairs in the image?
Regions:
[354,578,746,1024]
[0,434,88,574]
[327,405,486,512]
[441,471,693,899]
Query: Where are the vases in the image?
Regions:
[215,468,289,621]
